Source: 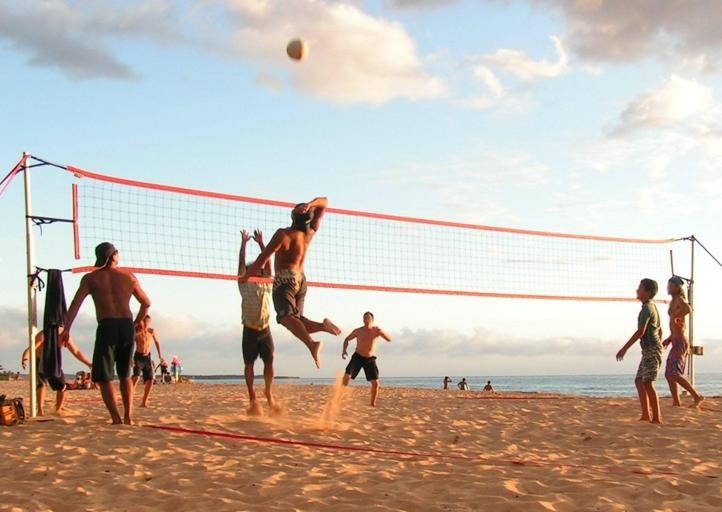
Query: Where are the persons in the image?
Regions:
[662,276,706,409]
[616,278,665,423]
[21,323,92,416]
[342,312,392,406]
[71,371,98,389]
[238,197,341,369]
[152,355,183,384]
[59,241,151,426]
[444,376,493,392]
[237,228,275,415]
[132,314,161,408]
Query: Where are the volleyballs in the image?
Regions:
[286,38,305,61]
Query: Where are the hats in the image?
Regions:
[293,201,314,234]
[91,241,119,270]
